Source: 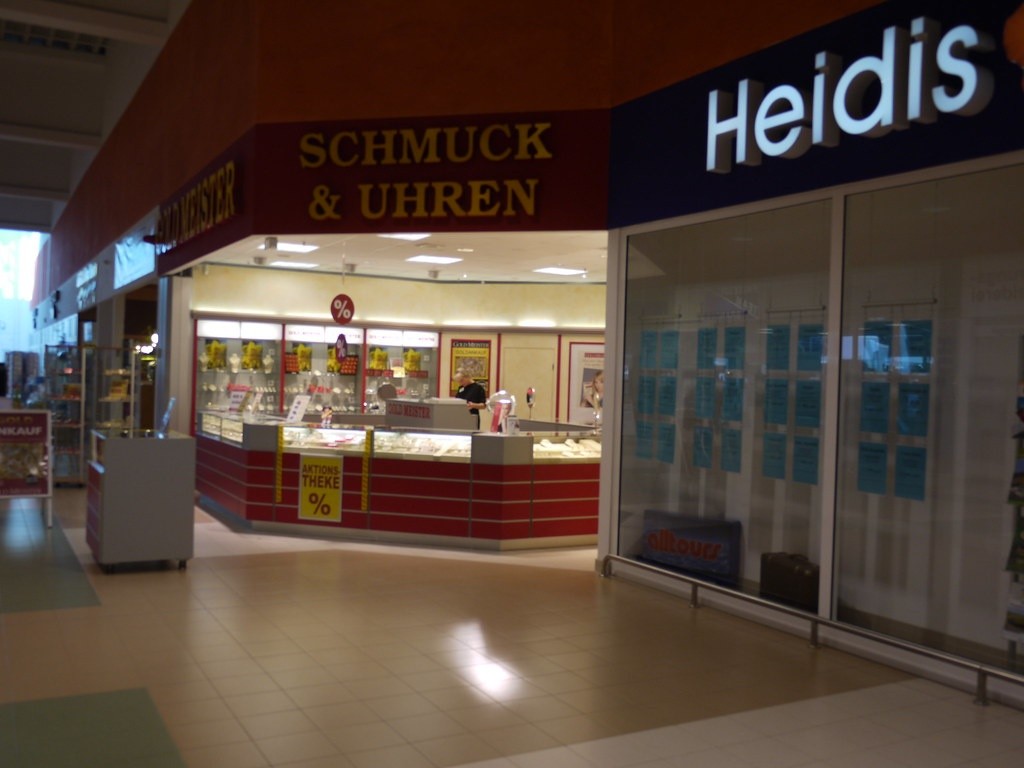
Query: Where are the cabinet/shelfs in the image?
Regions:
[16,343,137,487]
[87,428,195,573]
[190,317,441,436]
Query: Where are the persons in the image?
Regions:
[454,366,487,429]
[582,371,604,407]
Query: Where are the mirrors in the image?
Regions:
[485,390,516,434]
[592,393,601,436]
[526,387,536,419]
